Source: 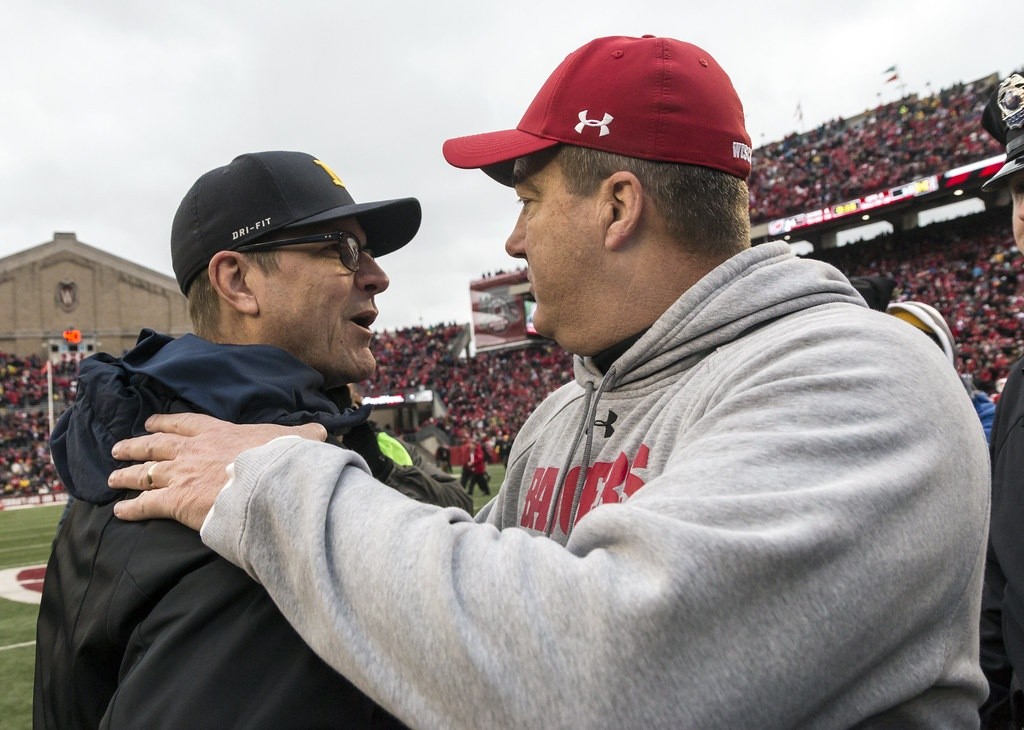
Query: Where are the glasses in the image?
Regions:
[442,34,753,188]
[208,230,375,294]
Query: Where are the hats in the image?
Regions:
[981,70,1024,193]
[171,150,421,298]
[887,301,957,367]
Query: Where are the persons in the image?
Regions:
[106,36,990,729]
[982,65,1023,727]
[748,80,1024,403]
[0,351,87,498]
[355,265,575,497]
[884,299,995,440]
[30,151,476,729]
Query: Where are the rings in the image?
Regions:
[147,461,160,489]
